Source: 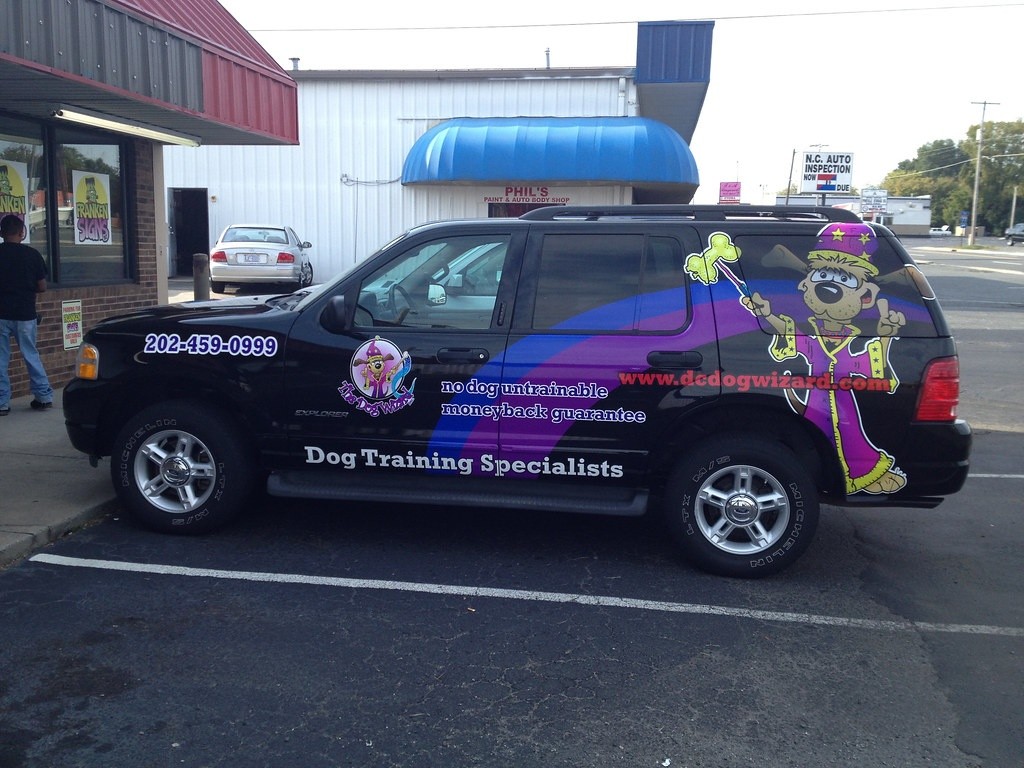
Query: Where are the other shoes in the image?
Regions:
[30,399,53,410]
[0,407,11,416]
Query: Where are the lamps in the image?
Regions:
[46,103,212,148]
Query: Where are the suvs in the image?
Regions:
[63,202,970,581]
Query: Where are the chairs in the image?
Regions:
[233,235,250,240]
[267,236,286,243]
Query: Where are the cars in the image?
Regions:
[1004,223,1024,246]
[202,223,316,293]
[930,225,952,237]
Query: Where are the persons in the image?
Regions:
[0,215,54,416]
